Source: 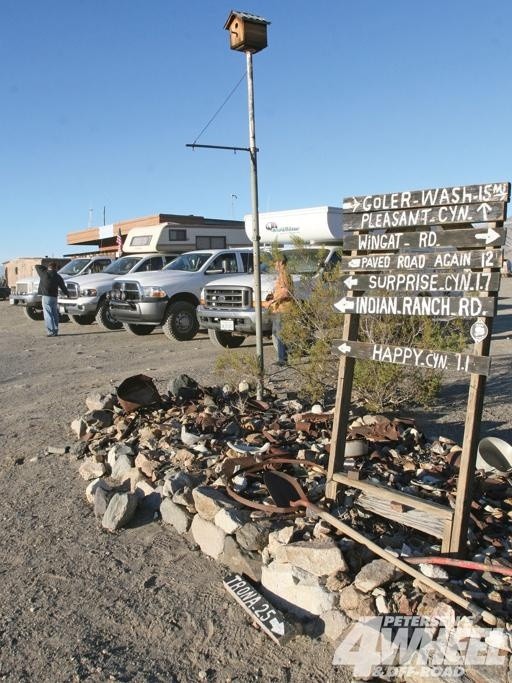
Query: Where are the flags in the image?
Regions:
[116,234,122,250]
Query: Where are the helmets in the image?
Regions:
[271,360,288,367]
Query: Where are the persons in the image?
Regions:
[35,262,69,337]
[265,254,294,366]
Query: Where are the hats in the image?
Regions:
[273,253,287,262]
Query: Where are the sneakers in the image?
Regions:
[46,329,60,337]
